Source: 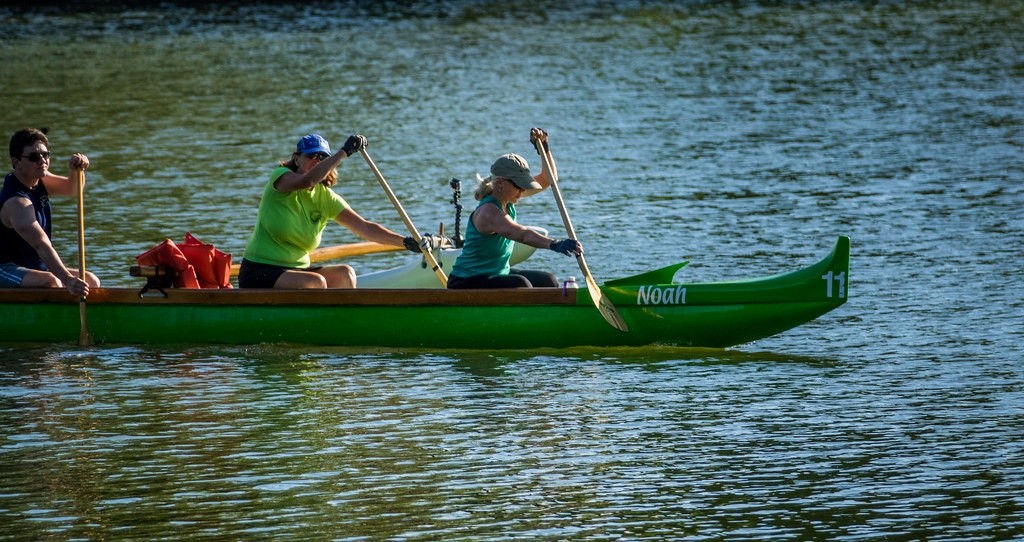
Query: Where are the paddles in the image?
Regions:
[354,142,448,289]
[534,127,629,333]
[69,160,90,347]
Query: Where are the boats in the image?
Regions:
[0,230,850,349]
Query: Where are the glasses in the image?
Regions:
[306,152,329,161]
[502,178,523,190]
[23,152,50,163]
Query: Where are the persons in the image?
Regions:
[237,134,433,290]
[0,128,99,299]
[446,127,584,289]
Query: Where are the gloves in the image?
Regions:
[341,135,368,157]
[403,233,430,252]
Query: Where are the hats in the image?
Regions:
[490,152,543,190]
[296,134,331,157]
